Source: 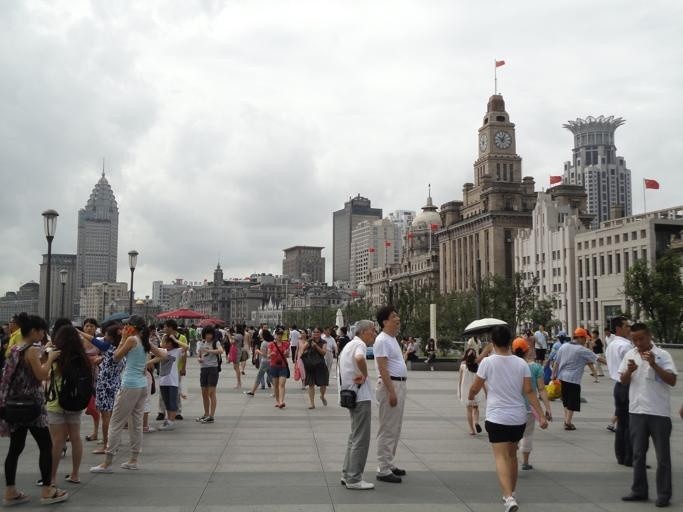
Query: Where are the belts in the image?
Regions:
[390,376,406,381]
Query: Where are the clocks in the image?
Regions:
[479,134,488,152]
[493,130,512,149]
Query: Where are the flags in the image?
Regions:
[385,242,390,247]
[407,233,412,239]
[431,224,439,230]
[643,178,659,190]
[549,175,561,184]
[368,247,374,252]
[495,60,504,67]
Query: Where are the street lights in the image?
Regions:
[127,250,138,314]
[388,279,393,304]
[144,294,149,323]
[58,268,68,318]
[41,209,59,335]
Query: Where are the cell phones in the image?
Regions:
[127,326,135,335]
[628,359,635,365]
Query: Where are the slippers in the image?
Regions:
[2,492,30,506]
[37,475,80,505]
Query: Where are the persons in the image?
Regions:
[466,325,548,509]
[193,322,351,422]
[401,336,437,371]
[606,316,678,507]
[0,312,191,504]
[337,306,407,488]
[459,324,615,470]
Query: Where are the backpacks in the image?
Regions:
[56,365,91,410]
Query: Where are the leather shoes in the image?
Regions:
[656,498,669,507]
[622,492,648,501]
[276,403,285,408]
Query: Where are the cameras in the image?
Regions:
[45,345,59,362]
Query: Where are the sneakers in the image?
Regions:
[522,464,532,470]
[121,462,137,470]
[86,434,105,454]
[341,478,374,489]
[607,425,616,431]
[476,424,482,433]
[143,413,183,432]
[564,423,576,430]
[90,464,113,473]
[196,415,214,424]
[320,396,327,405]
[503,496,519,512]
[377,468,405,483]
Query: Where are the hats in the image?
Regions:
[575,328,591,339]
[512,337,528,352]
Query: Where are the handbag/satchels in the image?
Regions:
[228,342,237,364]
[256,352,259,368]
[5,398,41,422]
[544,361,552,384]
[340,390,356,408]
[295,363,301,380]
